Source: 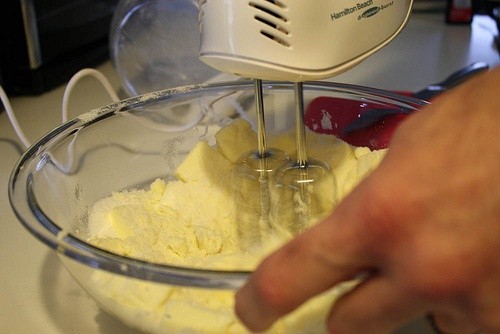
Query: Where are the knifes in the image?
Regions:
[342,61,490,140]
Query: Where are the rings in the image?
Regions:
[427,312,441,333]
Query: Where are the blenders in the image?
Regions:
[195,1,414,236]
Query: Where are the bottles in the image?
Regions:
[445,0,473,25]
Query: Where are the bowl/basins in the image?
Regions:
[7,80,434,334]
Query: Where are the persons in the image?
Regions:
[233,63,500,333]
